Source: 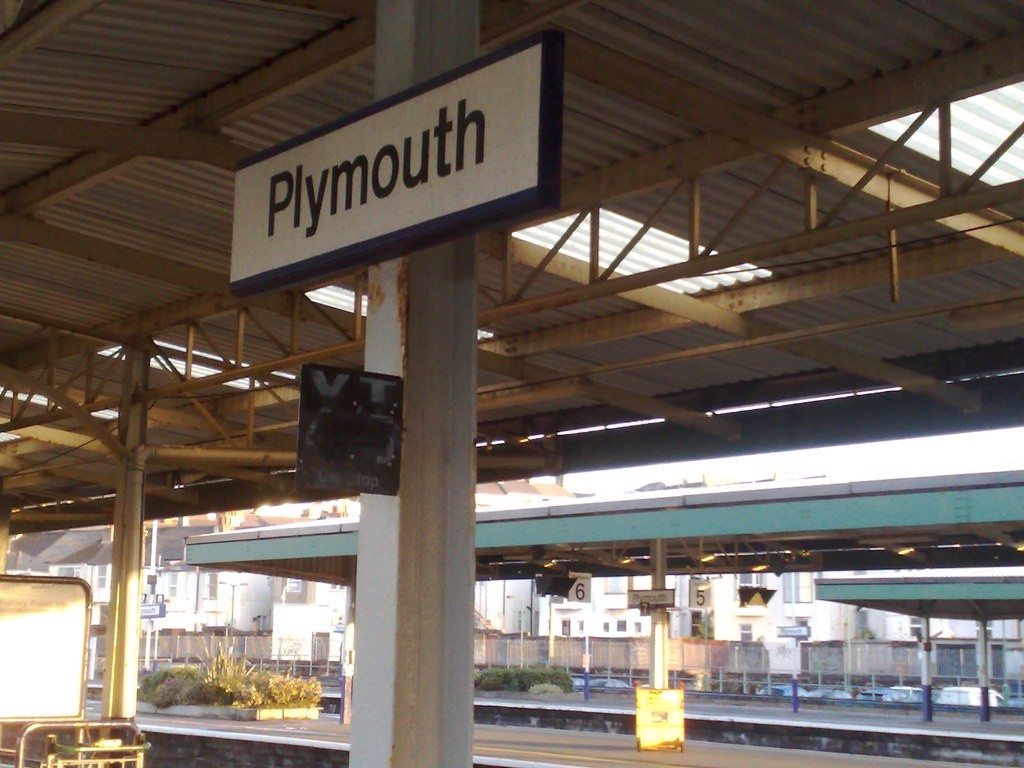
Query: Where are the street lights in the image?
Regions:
[507,593,524,669]
[218,580,248,649]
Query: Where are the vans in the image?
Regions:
[934,688,1004,707]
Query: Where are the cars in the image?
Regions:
[571,678,585,686]
[881,686,924,703]
[759,685,808,696]
[806,690,853,699]
[854,688,900,701]
[588,679,630,688]
